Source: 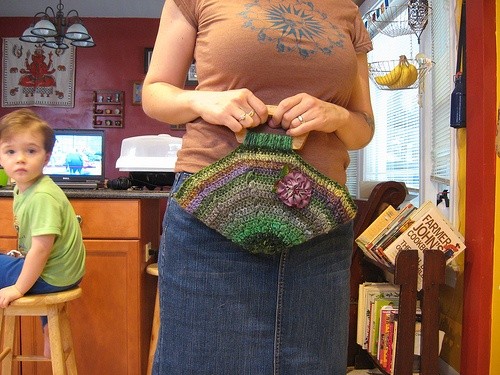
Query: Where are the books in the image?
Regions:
[355,200,466,292]
[357,281,445,375]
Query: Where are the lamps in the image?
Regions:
[18,0,95,49]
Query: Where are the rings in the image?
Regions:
[238,113,246,121]
[248,109,255,117]
[298,116,304,124]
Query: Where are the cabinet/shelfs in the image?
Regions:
[0,197,171,375]
[92,90,125,129]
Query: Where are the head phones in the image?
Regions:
[107,177,132,190]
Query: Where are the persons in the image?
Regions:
[141,0,374,375]
[0,108,87,359]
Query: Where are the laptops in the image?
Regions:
[42,128,105,189]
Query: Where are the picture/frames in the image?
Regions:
[184,58,199,86]
[133,82,141,105]
[144,48,153,75]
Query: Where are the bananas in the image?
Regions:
[374,55,418,88]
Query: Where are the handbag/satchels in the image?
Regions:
[171,105,358,255]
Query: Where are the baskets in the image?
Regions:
[365,5,434,37]
[368,59,436,91]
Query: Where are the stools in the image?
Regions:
[0,285,82,375]
[145,262,160,375]
